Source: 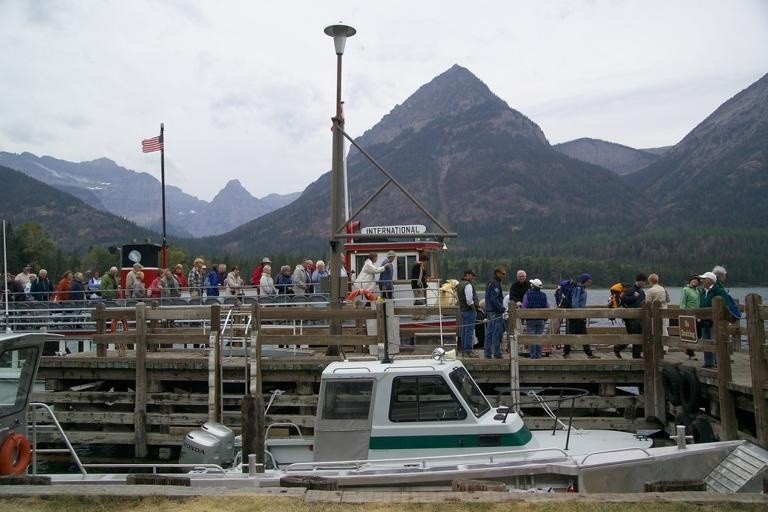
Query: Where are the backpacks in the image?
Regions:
[204,273,215,288]
[722,288,743,322]
[555,279,577,309]
[607,282,633,326]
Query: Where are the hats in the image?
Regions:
[464,269,476,276]
[529,278,543,288]
[133,262,144,267]
[24,264,32,270]
[636,273,648,282]
[698,272,718,282]
[688,274,700,283]
[386,250,396,258]
[580,273,592,284]
[261,258,272,264]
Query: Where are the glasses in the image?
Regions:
[500,271,506,275]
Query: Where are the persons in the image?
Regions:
[1,265,123,331]
[441,268,601,360]
[351,251,397,307]
[680,265,742,369]
[124,257,244,302]
[251,252,349,308]
[411,253,429,321]
[606,273,670,359]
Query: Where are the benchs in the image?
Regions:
[0,294,328,348]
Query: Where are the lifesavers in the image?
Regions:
[347,289,376,301]
[0,435,32,475]
[109,319,130,333]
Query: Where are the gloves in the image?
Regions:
[384,263,392,269]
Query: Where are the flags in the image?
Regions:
[141,136,163,153]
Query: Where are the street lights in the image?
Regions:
[316,22,361,307]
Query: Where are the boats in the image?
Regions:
[39,228,480,356]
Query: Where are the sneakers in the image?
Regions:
[464,353,504,358]
[688,355,718,369]
[632,355,645,359]
[587,354,601,359]
[562,352,571,359]
[614,346,622,359]
